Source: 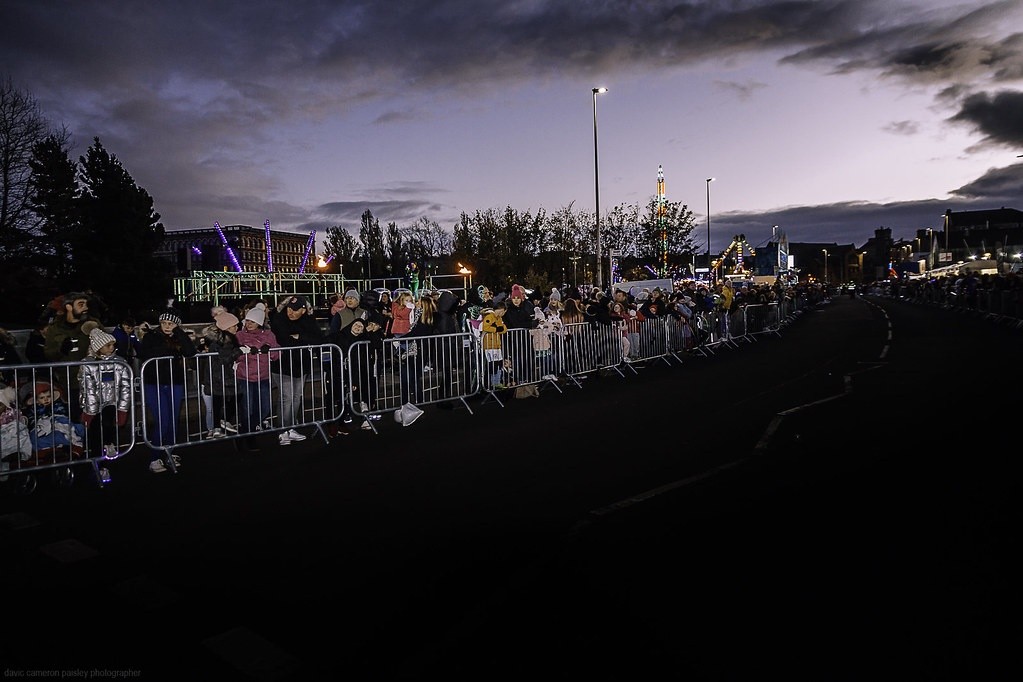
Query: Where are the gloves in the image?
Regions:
[239,344,251,354]
[172,327,181,333]
[60,337,77,356]
[250,346,259,355]
[261,345,271,353]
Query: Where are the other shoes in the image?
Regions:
[98,468,112,484]
[167,455,181,468]
[206,420,237,439]
[103,442,119,460]
[149,459,168,472]
[278,428,307,445]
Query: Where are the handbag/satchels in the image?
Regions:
[514,386,540,399]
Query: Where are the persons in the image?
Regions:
[889,266,1023,321]
[840,281,877,299]
[0,278,832,496]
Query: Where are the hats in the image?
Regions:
[550,287,559,300]
[287,296,306,311]
[211,305,239,330]
[512,284,521,298]
[345,290,360,302]
[158,308,183,326]
[588,288,707,307]
[81,321,116,353]
[246,302,266,326]
[63,291,91,304]
[0,386,17,415]
[534,307,546,321]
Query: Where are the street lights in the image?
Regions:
[822,248,828,285]
[706,178,711,280]
[940,214,949,252]
[926,227,933,270]
[906,244,912,253]
[914,237,921,260]
[592,84,608,291]
